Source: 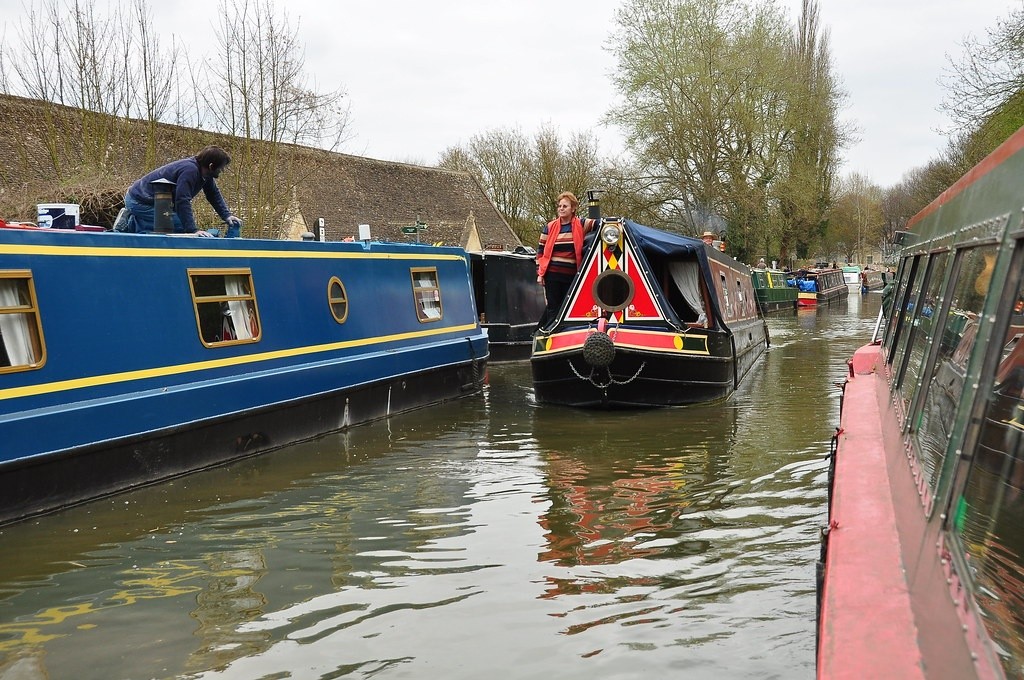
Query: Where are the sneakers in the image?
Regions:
[113,208,133,232]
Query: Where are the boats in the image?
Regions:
[0,213,491,526]
[750,258,894,315]
[529,189,770,407]
[468,243,548,365]
[816,124,1024,680]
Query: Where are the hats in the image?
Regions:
[699,231,718,240]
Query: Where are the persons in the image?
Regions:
[757,258,767,268]
[783,266,789,272]
[845,260,848,264]
[113,145,243,237]
[833,261,836,269]
[698,232,716,246]
[865,265,869,270]
[886,267,890,272]
[536,192,600,321]
